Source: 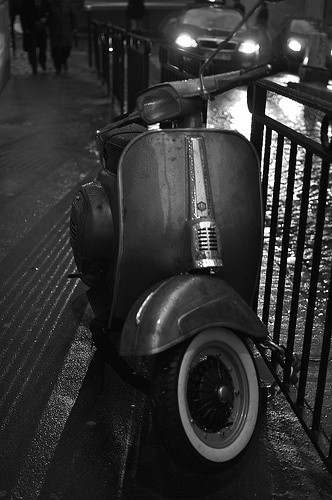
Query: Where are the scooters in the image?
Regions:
[67,61,275,467]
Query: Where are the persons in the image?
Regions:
[17,0,50,75]
[46,0,74,73]
[255,2,269,29]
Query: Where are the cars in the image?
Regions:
[160,6,263,81]
[274,14,331,80]
